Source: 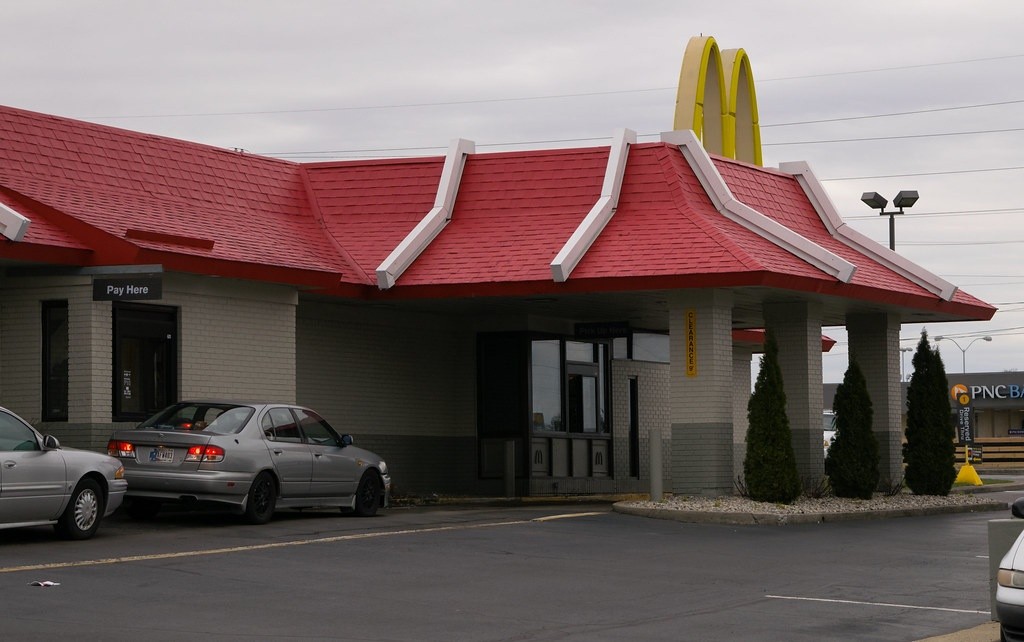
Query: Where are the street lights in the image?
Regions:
[861,190,919,251]
[901,346,913,382]
[934,335,992,373]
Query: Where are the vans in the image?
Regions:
[823,409,839,458]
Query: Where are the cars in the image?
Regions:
[0,406,129,540]
[997,498,1024,642]
[107,399,391,525]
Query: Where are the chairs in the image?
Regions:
[216,410,236,430]
[271,414,296,436]
